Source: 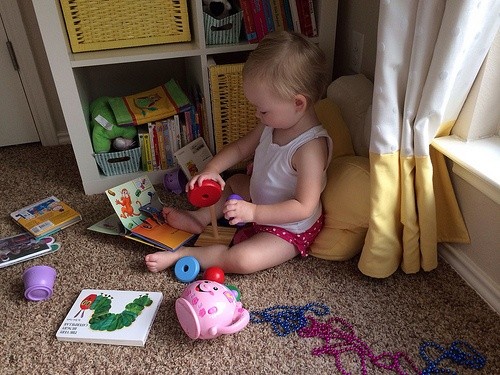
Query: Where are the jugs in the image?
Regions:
[174,278,250,340]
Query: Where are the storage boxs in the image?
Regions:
[203,11,242,44]
[92,146,142,176]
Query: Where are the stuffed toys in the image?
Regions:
[88,91,136,154]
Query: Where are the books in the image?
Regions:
[86,96,214,249]
[55,289,163,347]
[239,0,318,44]
[10,196,82,241]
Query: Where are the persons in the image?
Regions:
[145,28,332,275]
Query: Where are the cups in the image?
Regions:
[21,265,57,300]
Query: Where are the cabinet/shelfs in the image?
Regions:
[31,0,339,195]
